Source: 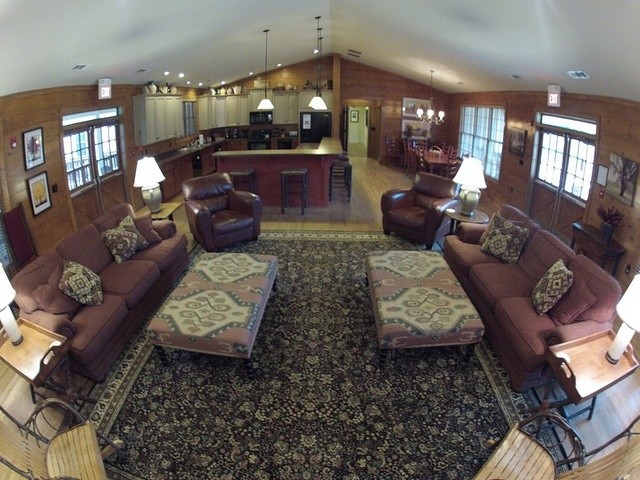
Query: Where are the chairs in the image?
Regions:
[413,141,427,149]
[418,147,430,173]
[403,140,410,171]
[472,411,640,479]
[0,398,124,480]
[440,156,460,177]
[385,136,402,166]
[442,144,451,157]
[449,146,458,159]
[409,149,421,177]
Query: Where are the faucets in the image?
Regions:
[191,139,199,148]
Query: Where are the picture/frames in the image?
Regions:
[596,164,608,187]
[402,96,433,120]
[605,152,639,207]
[22,127,45,172]
[509,127,527,157]
[401,120,432,141]
[26,171,52,216]
[350,110,359,122]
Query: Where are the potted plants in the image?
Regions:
[596,205,624,240]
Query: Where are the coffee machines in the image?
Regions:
[274,127,280,137]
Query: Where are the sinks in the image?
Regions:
[180,147,192,151]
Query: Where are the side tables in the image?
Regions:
[0,317,98,420]
[518,328,639,441]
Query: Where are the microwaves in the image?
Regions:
[249,112,272,124]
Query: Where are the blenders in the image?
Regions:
[223,127,231,138]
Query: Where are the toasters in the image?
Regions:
[289,130,298,137]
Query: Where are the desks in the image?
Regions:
[366,250,485,368]
[570,222,626,277]
[444,206,490,234]
[147,253,279,381]
[135,202,183,222]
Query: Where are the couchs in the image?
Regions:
[443,205,622,392]
[381,172,461,250]
[10,203,189,382]
[182,172,262,252]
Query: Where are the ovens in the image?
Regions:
[249,142,271,150]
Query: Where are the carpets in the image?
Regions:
[54,229,572,480]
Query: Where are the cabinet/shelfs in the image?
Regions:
[273,91,299,124]
[298,91,333,112]
[132,93,166,147]
[227,94,250,126]
[198,95,227,130]
[202,146,216,176]
[165,93,184,140]
[161,154,193,201]
[221,140,247,151]
[249,88,272,112]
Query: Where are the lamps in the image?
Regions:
[605,274,640,365]
[133,156,166,213]
[308,16,327,110]
[452,158,487,217]
[0,263,23,346]
[257,30,275,110]
[417,72,445,126]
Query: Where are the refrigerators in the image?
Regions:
[300,112,331,143]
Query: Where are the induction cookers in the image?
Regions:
[251,130,274,142]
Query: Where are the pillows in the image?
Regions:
[481,214,530,265]
[547,272,597,324]
[479,213,524,245]
[32,264,82,321]
[101,215,149,264]
[531,259,574,316]
[117,213,162,245]
[59,259,103,306]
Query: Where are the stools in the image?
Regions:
[229,168,256,194]
[343,151,347,155]
[280,168,309,216]
[334,157,349,161]
[329,161,352,202]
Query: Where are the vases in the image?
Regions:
[233,86,241,94]
[142,85,149,94]
[227,87,232,95]
[148,82,157,93]
[293,85,298,91]
[170,85,177,94]
[285,84,292,91]
[220,88,226,95]
[276,82,284,91]
